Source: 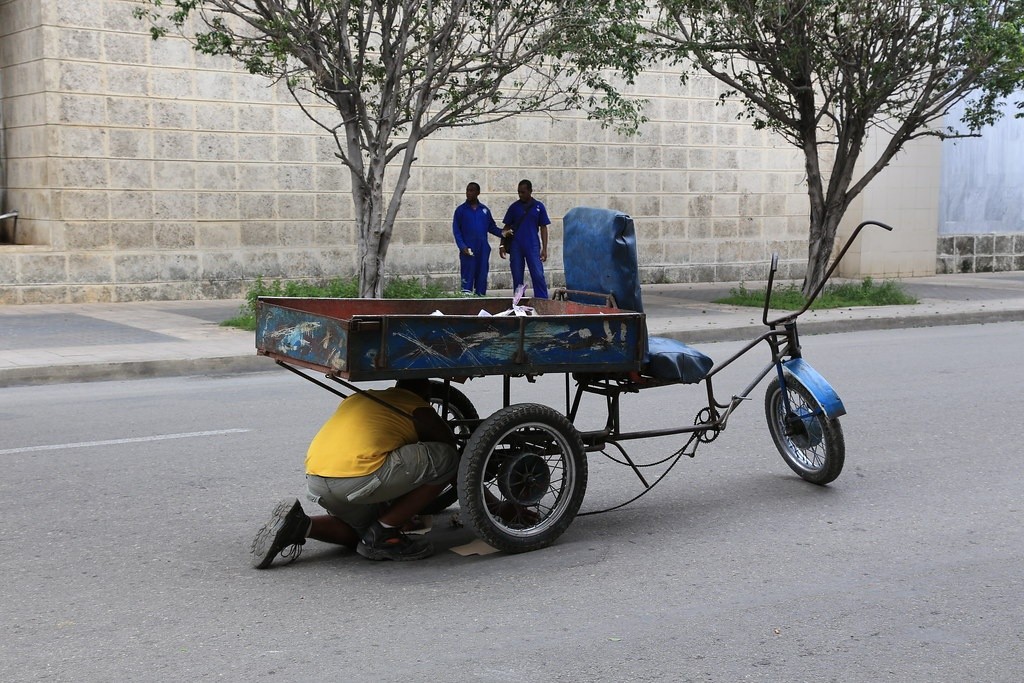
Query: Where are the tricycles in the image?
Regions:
[256,205,892,555]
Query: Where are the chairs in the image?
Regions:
[562,206,713,393]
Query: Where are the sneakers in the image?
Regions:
[250,496,310,569]
[356,519,433,560]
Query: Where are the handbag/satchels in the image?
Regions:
[504,227,515,255]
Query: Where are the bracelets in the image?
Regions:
[498,245,504,248]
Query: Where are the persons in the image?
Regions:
[499,179,549,299]
[453,181,514,297]
[248,377,460,577]
